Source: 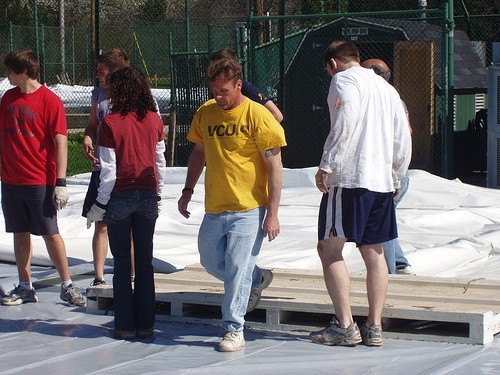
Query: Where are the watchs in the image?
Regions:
[182,188,193,193]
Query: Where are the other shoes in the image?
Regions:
[90,277,106,286]
[114,327,153,338]
[396,266,416,276]
[130,275,135,282]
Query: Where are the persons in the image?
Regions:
[87,68,166,340]
[179,58,287,352]
[310,42,411,346]
[0,49,86,308]
[81,49,412,313]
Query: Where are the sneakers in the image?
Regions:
[246,268,274,313]
[2,284,38,305]
[309,316,363,345]
[359,321,383,346]
[60,283,85,306]
[219,330,245,352]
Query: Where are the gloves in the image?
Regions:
[52,177,69,211]
[86,203,106,229]
